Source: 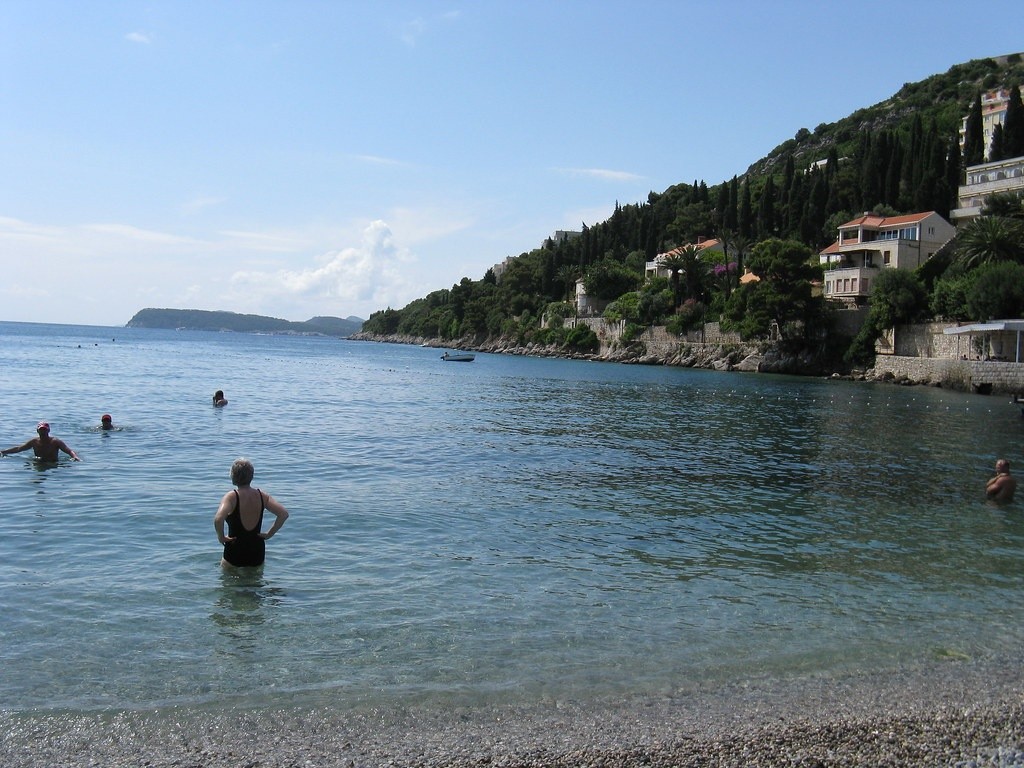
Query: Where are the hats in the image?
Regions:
[37,422,50,431]
[102,414,111,421]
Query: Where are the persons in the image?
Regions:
[214,458,289,568]
[212,391,227,405]
[986,459,1016,505]
[0,422,83,462]
[101,414,113,428]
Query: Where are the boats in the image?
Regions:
[440,353,476,362]
